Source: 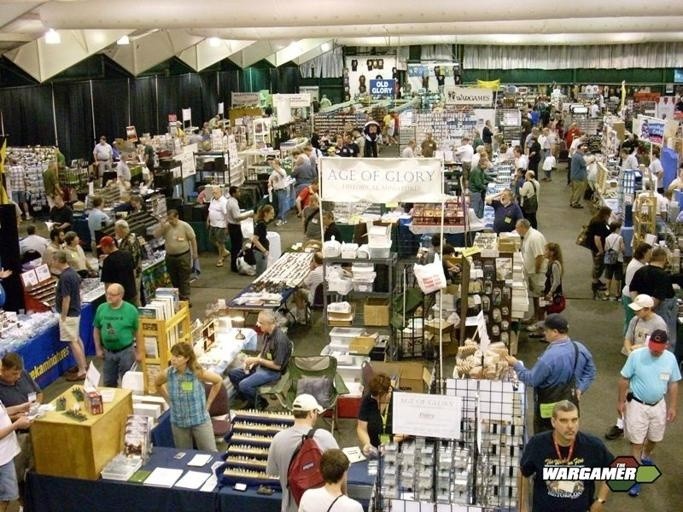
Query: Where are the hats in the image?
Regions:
[649,329,668,353]
[95,236,114,249]
[628,294,653,312]
[292,394,324,414]
[537,314,569,332]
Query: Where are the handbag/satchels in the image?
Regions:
[575,226,589,248]
[546,294,566,312]
[523,194,538,214]
[236,248,256,276]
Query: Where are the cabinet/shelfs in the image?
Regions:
[30,385,133,480]
[22,444,386,512]
[0,310,59,396]
[134,322,255,447]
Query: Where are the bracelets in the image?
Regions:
[596,497,606,504]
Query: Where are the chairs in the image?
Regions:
[205,383,232,443]
[255,340,295,412]
[274,355,340,442]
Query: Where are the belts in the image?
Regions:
[165,249,192,256]
[632,396,662,406]
[101,341,136,353]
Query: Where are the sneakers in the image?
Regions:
[522,318,548,342]
[606,424,625,439]
[641,456,652,465]
[592,280,621,297]
[216,253,231,259]
[65,373,87,382]
[570,203,583,208]
[629,482,642,497]
[216,257,224,267]
[68,363,90,372]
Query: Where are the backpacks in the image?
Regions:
[287,428,326,508]
[604,236,620,264]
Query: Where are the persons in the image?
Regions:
[154,342,224,452]
[0,400,34,511]
[520,402,616,512]
[266,393,339,511]
[505,312,598,435]
[228,308,291,411]
[605,294,669,440]
[0,354,43,506]
[0,74,682,397]
[297,449,364,512]
[619,330,682,497]
[357,375,411,459]
